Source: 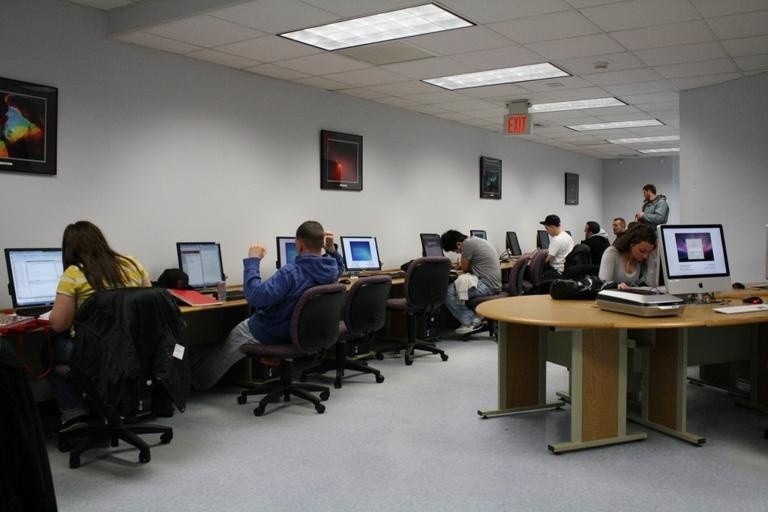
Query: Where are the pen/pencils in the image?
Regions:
[744,288,760,291]
[710,299,732,303]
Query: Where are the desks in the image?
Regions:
[472,285,766,466]
[1,256,464,454]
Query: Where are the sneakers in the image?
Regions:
[56,414,88,433]
[455,319,484,334]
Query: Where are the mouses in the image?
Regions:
[339,280,351,284]
[732,283,745,289]
[743,297,763,304]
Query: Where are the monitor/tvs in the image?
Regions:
[420,233,444,257]
[656,223,731,303]
[4,247,65,308]
[175,242,225,295]
[536,230,571,249]
[506,232,522,258]
[470,230,487,240]
[340,236,381,278]
[276,236,298,270]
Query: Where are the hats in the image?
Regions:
[539,214,561,225]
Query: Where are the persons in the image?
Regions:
[584,221,608,240]
[195,220,346,392]
[598,224,658,290]
[441,230,502,334]
[613,218,626,243]
[540,214,575,275]
[49,220,152,433]
[635,184,670,231]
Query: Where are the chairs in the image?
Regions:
[234,283,350,419]
[464,241,602,351]
[310,273,393,391]
[375,255,455,365]
[58,286,189,470]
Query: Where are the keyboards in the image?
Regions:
[213,290,245,301]
[358,271,406,279]
[16,304,53,317]
[712,304,768,314]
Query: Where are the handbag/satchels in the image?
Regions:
[549,271,615,298]
[1,313,53,379]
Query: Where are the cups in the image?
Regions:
[216,280,227,300]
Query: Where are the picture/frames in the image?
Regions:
[479,155,502,201]
[565,172,579,205]
[321,128,364,192]
[0,75,58,177]
